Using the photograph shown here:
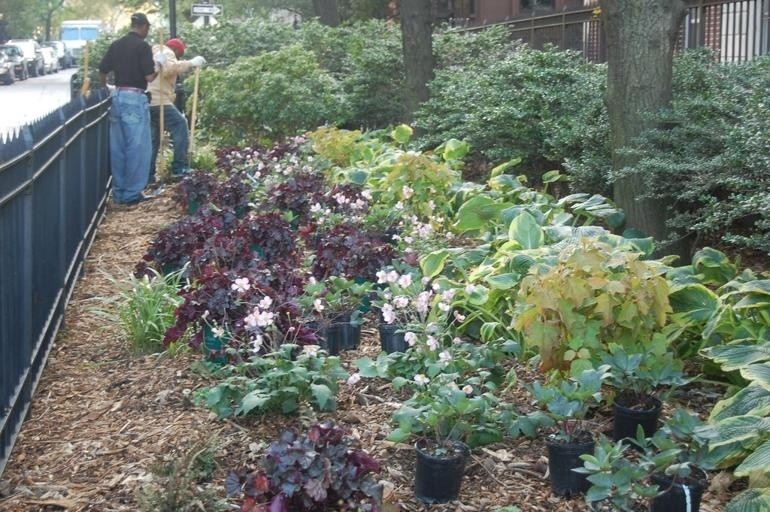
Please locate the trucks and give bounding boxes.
[57,19,104,69]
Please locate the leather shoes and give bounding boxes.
[128,191,154,205]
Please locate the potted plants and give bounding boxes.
[154,173,734,511]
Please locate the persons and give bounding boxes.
[145,38,207,184]
[99,13,167,206]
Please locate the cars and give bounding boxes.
[0,35,68,85]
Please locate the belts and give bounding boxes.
[115,87,146,94]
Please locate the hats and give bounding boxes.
[131,13,151,25]
[166,39,184,56]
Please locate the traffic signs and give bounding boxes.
[192,4,223,17]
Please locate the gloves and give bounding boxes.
[190,56,206,66]
[157,54,166,64]
